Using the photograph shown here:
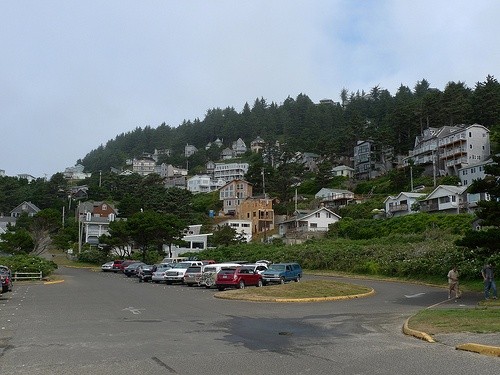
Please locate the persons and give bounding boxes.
[151,265,157,283]
[481,260,499,301]
[0,271,5,295]
[447,266,460,298]
[138,265,144,283]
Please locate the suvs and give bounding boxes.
[215,266,263,291]
[261,262,303,286]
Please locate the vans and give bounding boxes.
[202,263,240,289]
[163,260,203,285]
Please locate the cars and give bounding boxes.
[183,264,208,286]
[159,257,273,276]
[151,266,172,284]
[101,259,157,282]
